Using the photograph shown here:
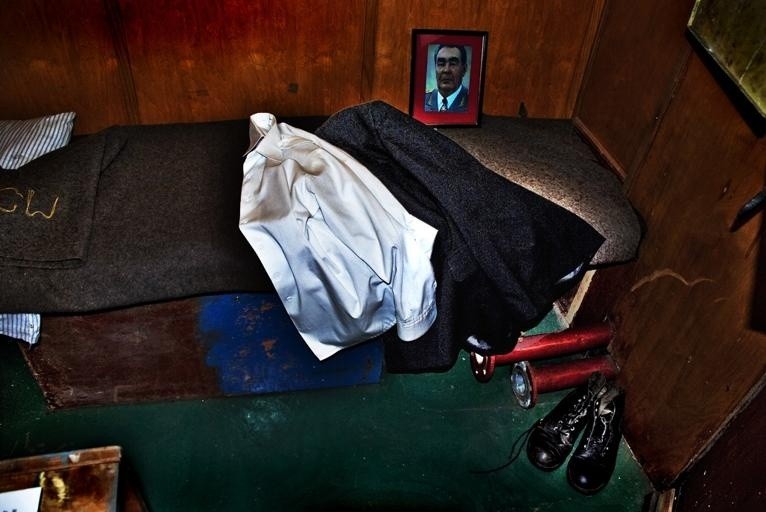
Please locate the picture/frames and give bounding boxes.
[409,26,487,128]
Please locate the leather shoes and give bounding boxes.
[567,383,626,494]
[527,370,606,471]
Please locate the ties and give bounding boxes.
[440,99,448,112]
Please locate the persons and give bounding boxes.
[423,43,470,114]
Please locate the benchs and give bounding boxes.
[1,116,644,328]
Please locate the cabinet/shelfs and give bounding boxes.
[0,446,150,512]
